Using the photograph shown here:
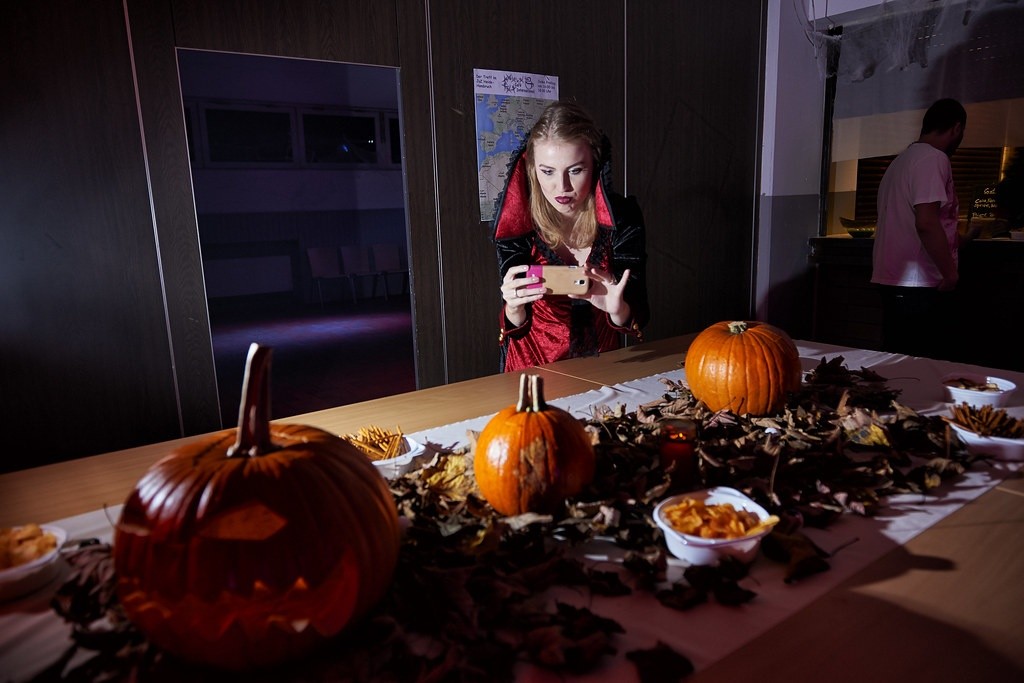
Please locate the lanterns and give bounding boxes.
[109,341,400,672]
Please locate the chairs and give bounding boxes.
[371,242,409,303]
[308,246,356,315]
[342,245,387,309]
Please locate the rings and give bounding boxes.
[514,288,519,298]
[609,273,617,285]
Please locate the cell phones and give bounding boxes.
[525,265,590,295]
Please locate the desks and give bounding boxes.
[0,332,1023,683]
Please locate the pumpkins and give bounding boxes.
[685,319,804,417]
[474,372,596,519]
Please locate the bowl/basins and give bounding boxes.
[0,526,68,593]
[371,436,427,480]
[655,485,771,568]
[938,372,1016,408]
[950,422,1024,462]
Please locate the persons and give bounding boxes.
[859,96,967,353]
[489,101,650,371]
